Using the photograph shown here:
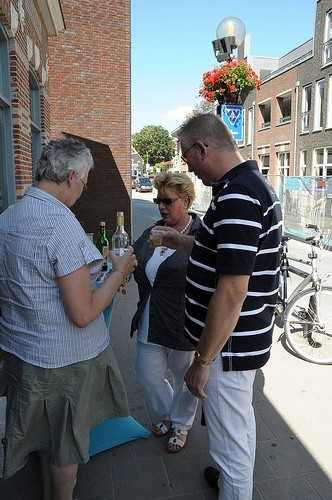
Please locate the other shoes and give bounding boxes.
[204,466,219,492]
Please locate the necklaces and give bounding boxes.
[160,215,192,256]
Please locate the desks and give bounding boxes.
[87,263,150,456]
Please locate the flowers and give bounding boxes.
[195,59,261,105]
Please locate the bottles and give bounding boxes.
[112,211,130,283]
[95,222,109,270]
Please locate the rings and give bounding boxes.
[134,261,137,266]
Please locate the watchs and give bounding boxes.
[194,350,214,365]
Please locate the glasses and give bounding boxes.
[153,197,178,205]
[180,141,208,163]
[80,179,88,193]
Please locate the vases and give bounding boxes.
[214,86,254,105]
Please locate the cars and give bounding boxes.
[131,175,138,188]
[136,177,154,192]
[148,176,155,182]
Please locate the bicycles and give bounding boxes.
[277,224,332,365]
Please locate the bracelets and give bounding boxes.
[112,269,126,282]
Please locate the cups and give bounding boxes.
[153,229,162,245]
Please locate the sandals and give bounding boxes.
[167,428,188,452]
[154,416,172,435]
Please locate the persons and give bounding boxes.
[146,115,283,500]
[131,173,202,451]
[0,138,137,500]
